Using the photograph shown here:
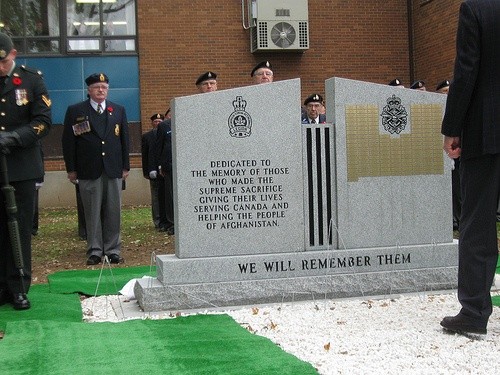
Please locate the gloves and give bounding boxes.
[35,182,43,190]
[150,170,157,178]
[0,131,21,147]
[72,180,78,184]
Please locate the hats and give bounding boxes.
[150,114,164,122]
[196,71,217,86]
[251,61,272,78]
[86,73,108,86]
[0,33,13,60]
[304,94,324,106]
[165,108,171,116]
[436,80,450,91]
[389,79,403,86]
[410,80,425,89]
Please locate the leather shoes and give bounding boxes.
[11,293,30,310]
[109,253,120,264]
[87,254,101,265]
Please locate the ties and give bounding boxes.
[0,75,9,89]
[97,104,103,114]
[311,120,316,124]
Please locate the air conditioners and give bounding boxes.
[247,0,309,52]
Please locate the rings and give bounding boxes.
[446,153,449,156]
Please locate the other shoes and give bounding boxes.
[168,229,174,235]
[159,225,167,231]
[80,232,87,240]
[32,228,38,236]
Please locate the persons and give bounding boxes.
[251,60,273,84]
[32,20,43,50]
[301,94,326,123]
[62,74,131,264]
[194,72,219,93]
[388,78,404,87]
[77,90,173,237]
[0,33,51,311]
[410,81,426,90]
[435,80,450,93]
[441,0,500,334]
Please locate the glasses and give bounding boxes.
[200,81,217,88]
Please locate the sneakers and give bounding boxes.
[441,312,488,331]
